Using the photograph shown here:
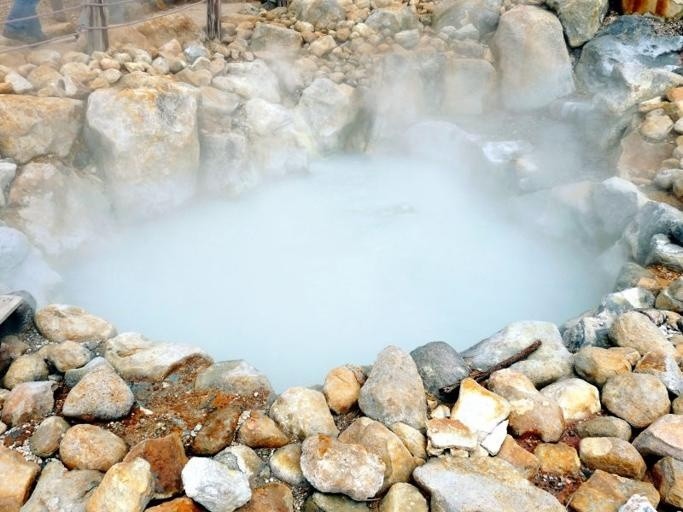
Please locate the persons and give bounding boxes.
[1,0,50,43]
